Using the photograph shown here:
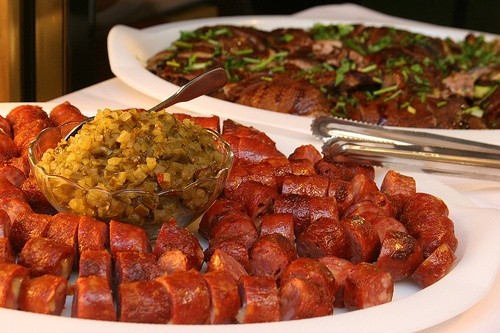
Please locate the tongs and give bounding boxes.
[310,114,500,170]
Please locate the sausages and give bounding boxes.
[0,100,458,325]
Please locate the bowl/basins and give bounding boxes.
[27,118,234,242]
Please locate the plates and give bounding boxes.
[0,109,499,332]
[107,2,500,149]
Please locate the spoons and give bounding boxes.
[62,68,228,144]
